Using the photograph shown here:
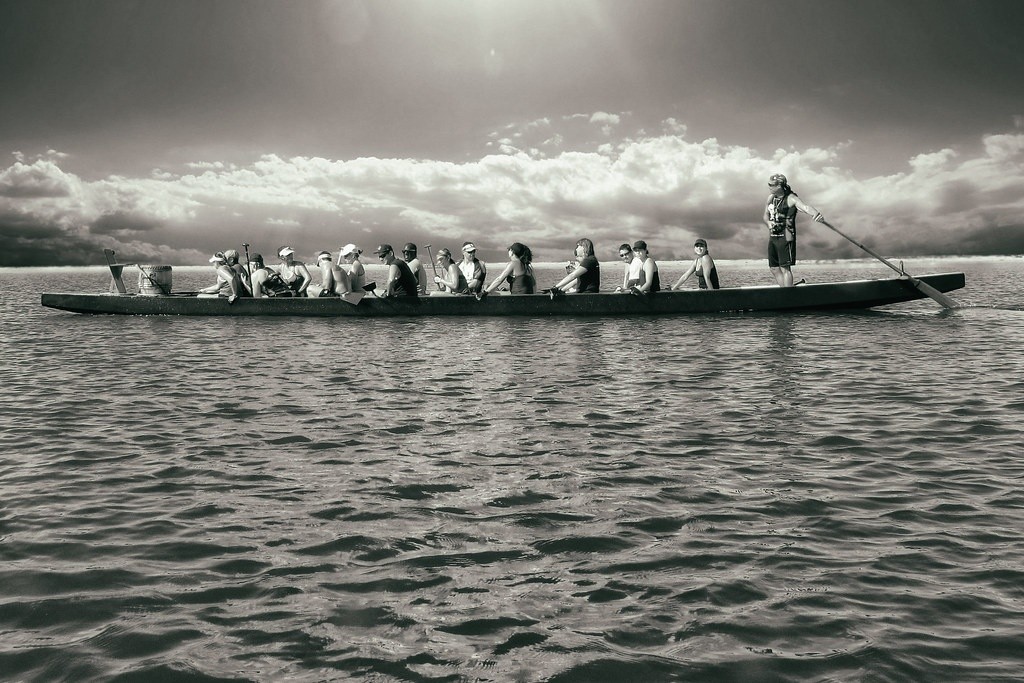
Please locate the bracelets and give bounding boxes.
[439,278,441,282]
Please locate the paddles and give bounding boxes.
[423,244,441,291]
[821,219,959,309]
[242,242,253,296]
[326,289,365,306]
[615,286,646,297]
[361,282,377,297]
[793,279,805,285]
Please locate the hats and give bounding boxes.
[245,253,263,263]
[340,244,360,257]
[209,254,224,263]
[373,244,393,253]
[316,254,332,267]
[462,244,477,253]
[693,238,707,245]
[225,250,239,259]
[632,241,646,250]
[279,247,297,258]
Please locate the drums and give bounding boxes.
[138,263,172,294]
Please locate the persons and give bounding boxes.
[541,238,660,296]
[433,241,537,301]
[671,239,719,290]
[763,173,824,288]
[196,243,427,304]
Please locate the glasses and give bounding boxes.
[379,252,389,259]
[768,183,776,187]
[401,249,415,255]
[620,252,630,257]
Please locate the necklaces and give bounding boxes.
[773,196,783,212]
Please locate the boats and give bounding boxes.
[40,248,967,317]
